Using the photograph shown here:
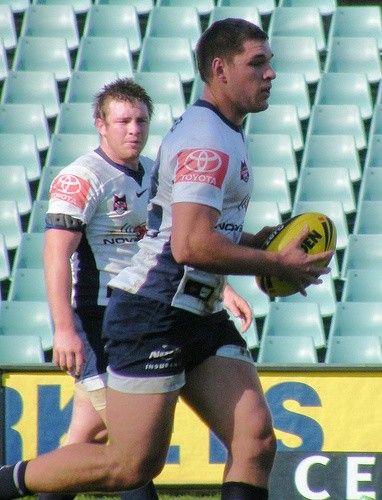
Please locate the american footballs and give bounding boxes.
[255,212,337,297]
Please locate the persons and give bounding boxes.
[0,18,335,500]
[38,78,253,500]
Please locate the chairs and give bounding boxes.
[0,0,382,367]
